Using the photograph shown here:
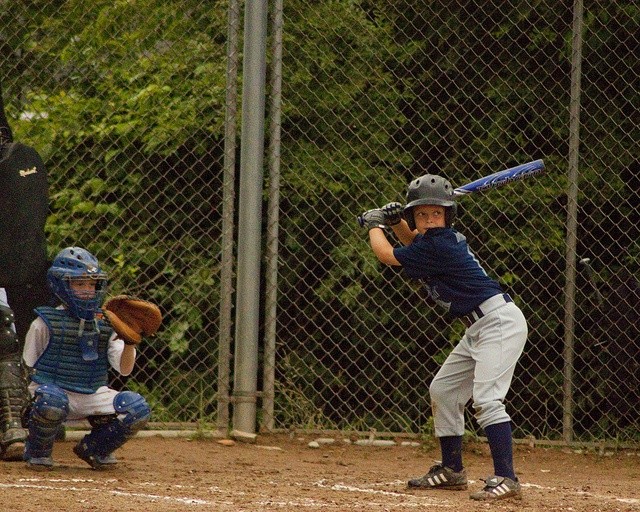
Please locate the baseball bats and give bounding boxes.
[357,158,546,228]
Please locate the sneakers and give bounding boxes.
[0,441,26,459]
[26,455,53,472]
[468,474,521,501]
[73,440,120,471]
[408,464,468,491]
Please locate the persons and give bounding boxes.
[0,137,66,441]
[22,246,148,470]
[360,176,529,500]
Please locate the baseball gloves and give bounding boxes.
[101,294,163,345]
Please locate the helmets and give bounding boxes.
[403,173,458,232]
[46,246,107,321]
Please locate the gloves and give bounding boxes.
[361,207,386,233]
[381,201,404,226]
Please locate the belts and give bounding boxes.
[459,292,513,328]
